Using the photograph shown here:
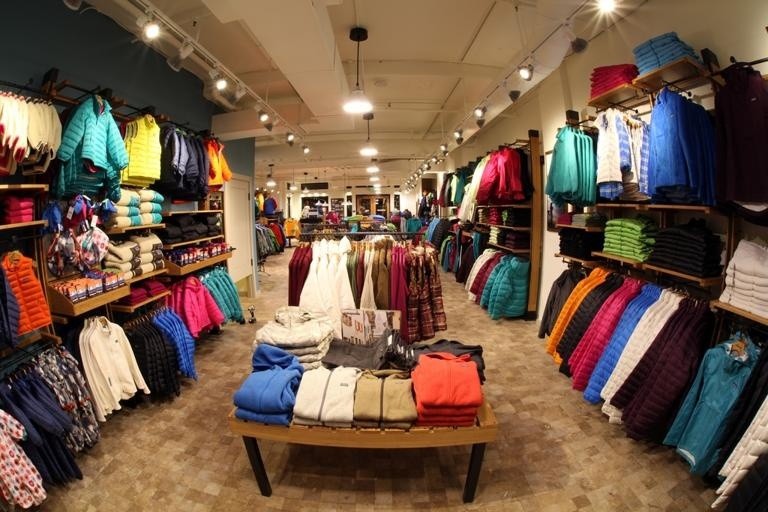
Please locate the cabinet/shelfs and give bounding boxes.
[633,56,712,94]
[94,209,233,314]
[474,204,530,255]
[587,81,647,109]
[548,204,730,287]
[0,178,52,234]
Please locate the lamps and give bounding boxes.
[342,25,372,116]
[358,114,377,157]
[135,8,160,39]
[454,126,464,145]
[440,144,449,157]
[178,38,195,60]
[475,106,487,129]
[507,63,536,105]
[253,105,280,133]
[563,25,588,53]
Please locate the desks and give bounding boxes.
[226,389,499,503]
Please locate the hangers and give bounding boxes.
[654,81,695,106]
[598,103,641,125]
[555,123,597,139]
